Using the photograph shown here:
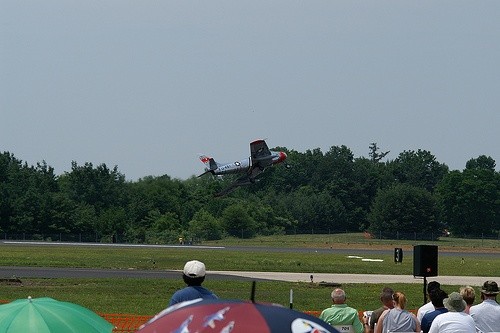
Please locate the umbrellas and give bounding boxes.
[0,294,114,333]
[136,299,340,333]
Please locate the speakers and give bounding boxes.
[413,245,438,276]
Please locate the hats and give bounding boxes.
[443,291,467,311]
[481,281,500,295]
[182,260,206,278]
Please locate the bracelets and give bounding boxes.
[363,323,368,328]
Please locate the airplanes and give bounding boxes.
[195,138,288,199]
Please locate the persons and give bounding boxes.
[319,288,370,333]
[370,288,394,333]
[470,280,500,333]
[169,259,218,307]
[421,290,449,333]
[377,292,421,333]
[459,286,476,313]
[428,292,478,333]
[416,281,440,333]
